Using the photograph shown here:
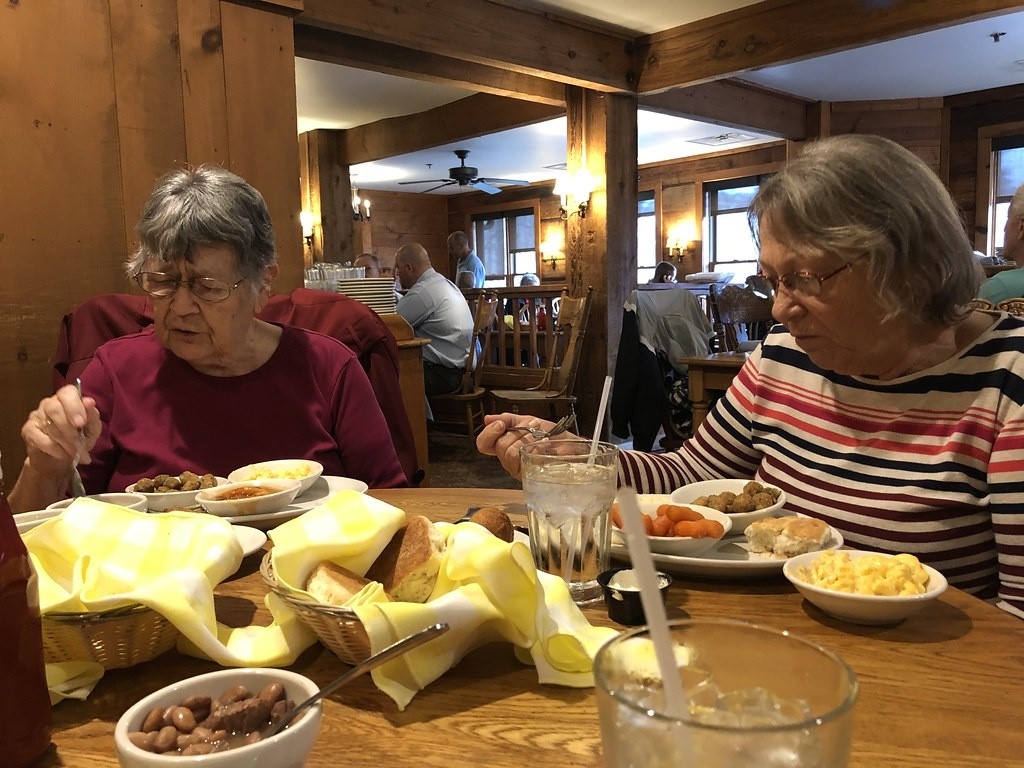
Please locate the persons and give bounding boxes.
[504,272,543,320]
[477,133,1024,619]
[392,243,484,392]
[382,267,402,290]
[0,163,407,516]
[456,270,478,309]
[975,184,1024,304]
[646,260,678,285]
[354,253,404,304]
[447,230,485,289]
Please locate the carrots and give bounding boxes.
[611,502,724,539]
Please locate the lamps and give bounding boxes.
[543,250,565,269]
[300,212,316,248]
[667,232,688,261]
[553,171,597,219]
[351,188,371,221]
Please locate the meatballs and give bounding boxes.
[692,481,781,511]
[133,471,218,493]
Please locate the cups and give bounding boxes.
[597,565,672,624]
[519,439,620,607]
[592,616,858,768]
[304,262,366,291]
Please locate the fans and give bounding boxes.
[398,150,534,195]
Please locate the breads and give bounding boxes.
[742,515,832,557]
[304,513,448,608]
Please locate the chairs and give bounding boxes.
[50,286,413,489]
[490,283,596,438]
[709,280,773,351]
[431,291,502,442]
[611,288,716,455]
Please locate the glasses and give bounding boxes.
[132,258,252,301]
[745,250,873,300]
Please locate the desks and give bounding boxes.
[12,486,1024,768]
[395,338,435,474]
[675,350,752,440]
[478,328,569,368]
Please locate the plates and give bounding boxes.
[217,475,368,530]
[595,494,845,581]
[512,530,530,548]
[232,525,267,557]
[335,277,397,315]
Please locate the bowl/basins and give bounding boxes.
[46,492,148,513]
[113,667,322,768]
[194,477,302,516]
[671,478,788,534]
[229,459,324,497]
[612,502,733,558]
[782,549,949,625]
[13,508,66,533]
[125,475,229,511]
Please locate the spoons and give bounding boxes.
[71,377,85,498]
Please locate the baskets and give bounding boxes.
[261,548,371,667]
[40,602,174,671]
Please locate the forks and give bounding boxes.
[505,414,577,439]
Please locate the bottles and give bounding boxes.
[536,307,547,330]
[0,461,61,768]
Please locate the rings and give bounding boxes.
[40,420,54,430]
